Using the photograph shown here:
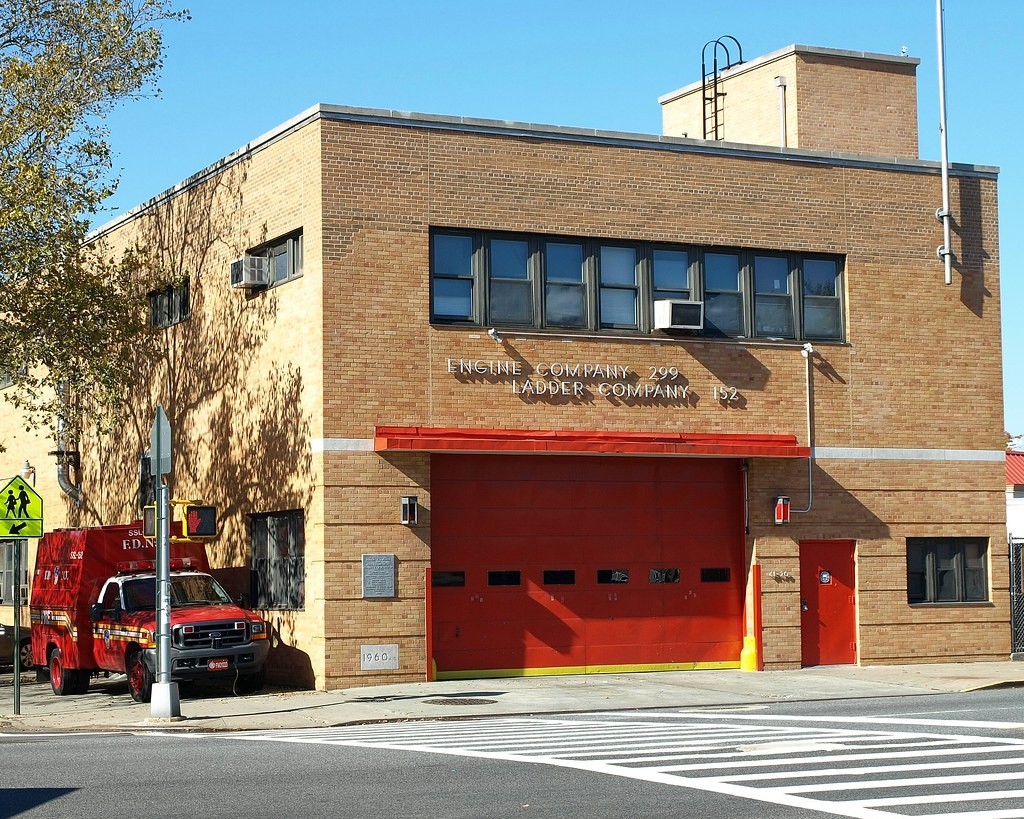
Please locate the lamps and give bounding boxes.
[22,460,35,479]
[401,493,418,524]
[774,495,791,525]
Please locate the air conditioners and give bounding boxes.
[231,256,268,287]
[654,298,704,329]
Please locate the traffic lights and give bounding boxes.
[181,505,218,540]
[142,501,162,540]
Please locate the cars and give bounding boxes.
[0,623,37,671]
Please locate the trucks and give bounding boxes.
[28,520,272,704]
